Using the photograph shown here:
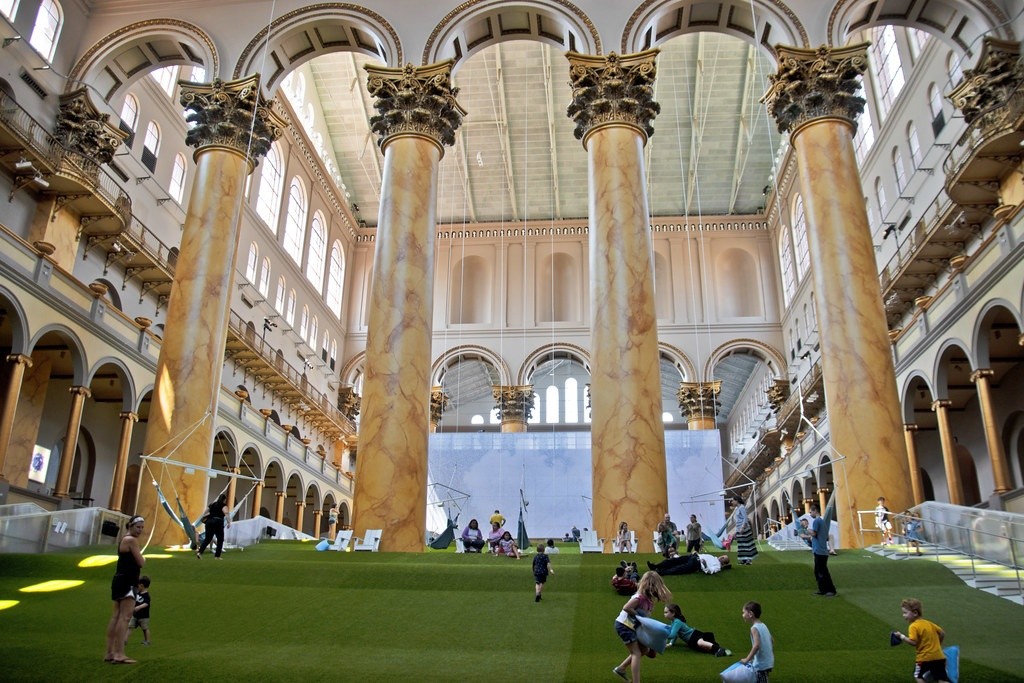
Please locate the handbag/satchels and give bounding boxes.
[201,512,210,524]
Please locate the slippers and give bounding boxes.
[104,657,136,665]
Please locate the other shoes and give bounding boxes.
[535,592,541,602]
[140,640,151,646]
[829,551,837,556]
[814,592,837,597]
[197,553,202,559]
[620,560,637,572]
[214,557,224,560]
[646,560,657,571]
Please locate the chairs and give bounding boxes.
[453,528,477,554]
[320,530,353,552]
[650,531,663,553]
[351,529,382,552]
[612,531,639,554]
[578,531,606,554]
[487,541,492,552]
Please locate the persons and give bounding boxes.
[733,495,759,565]
[657,514,680,558]
[124,575,151,646]
[739,601,774,683]
[647,551,729,575]
[806,504,837,597]
[104,516,145,664]
[663,604,732,658]
[875,497,895,546]
[571,526,580,542]
[544,539,559,554]
[532,544,555,602]
[490,510,506,528]
[612,561,640,596]
[195,494,230,560]
[798,518,838,557]
[498,531,524,560]
[488,522,505,554]
[901,509,926,558]
[686,514,702,553]
[894,598,951,683]
[462,518,485,553]
[612,571,671,683]
[617,522,632,553]
[327,503,340,541]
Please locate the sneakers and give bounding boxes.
[613,667,629,682]
[715,647,732,658]
[738,562,752,566]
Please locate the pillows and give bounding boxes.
[315,539,329,551]
[922,644,961,683]
[635,615,672,655]
[720,660,759,683]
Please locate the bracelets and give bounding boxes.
[899,634,906,641]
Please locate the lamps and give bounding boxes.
[331,165,359,214]
[882,225,896,239]
[763,140,787,196]
[801,351,810,360]
